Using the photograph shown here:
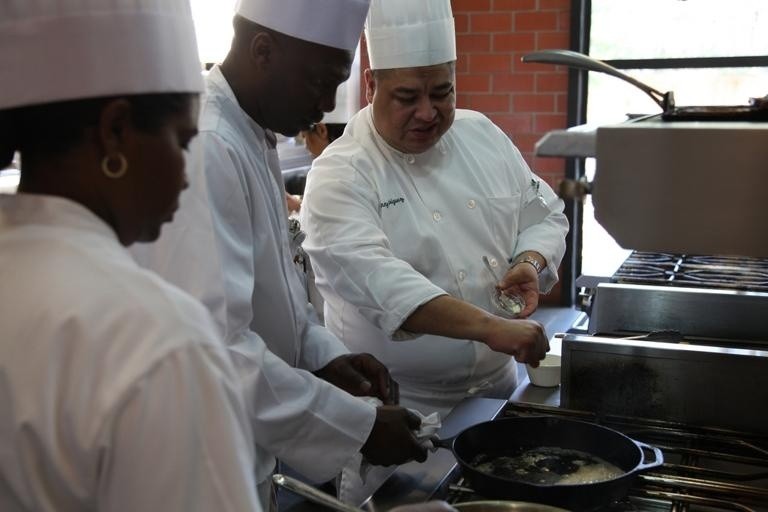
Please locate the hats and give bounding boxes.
[235,0,368,53]
[0,0,208,114]
[362,0,457,72]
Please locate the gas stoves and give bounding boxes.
[432,395,768,510]
[609,249,766,294]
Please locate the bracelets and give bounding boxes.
[507,256,543,274]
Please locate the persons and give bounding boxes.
[119,10,429,512]
[295,61,574,507]
[285,122,347,218]
[1,91,264,512]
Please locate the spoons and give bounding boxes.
[481,250,527,320]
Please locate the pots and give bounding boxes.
[384,411,664,502]
[520,41,764,118]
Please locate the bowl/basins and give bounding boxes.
[524,349,565,386]
